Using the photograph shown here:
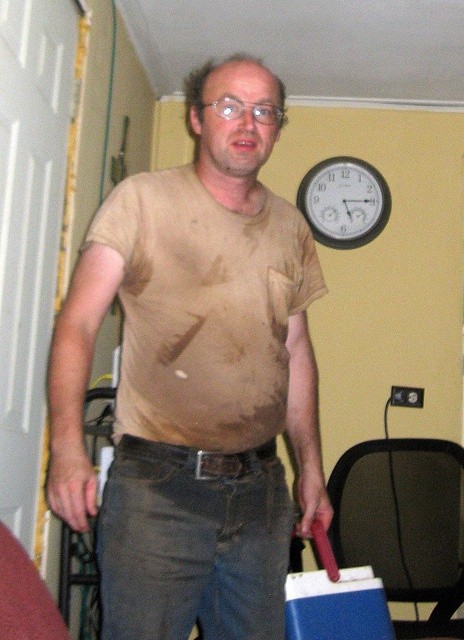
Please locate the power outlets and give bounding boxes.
[390,386,425,408]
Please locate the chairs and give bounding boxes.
[324,439,463,639]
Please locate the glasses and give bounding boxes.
[202,94,287,125]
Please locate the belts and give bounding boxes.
[116,433,276,483]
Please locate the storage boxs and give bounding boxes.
[279,514,398,638]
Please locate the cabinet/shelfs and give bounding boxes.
[57,386,119,640]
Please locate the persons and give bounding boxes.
[44,54,333,639]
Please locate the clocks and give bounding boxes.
[298,156,392,249]
